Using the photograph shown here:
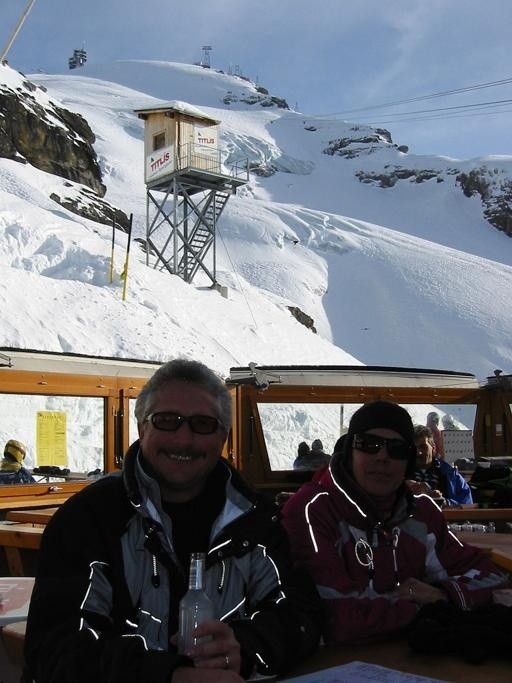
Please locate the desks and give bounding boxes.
[275,634,512,683]
[0,506,60,673]
[440,506,512,572]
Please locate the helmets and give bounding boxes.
[4,439,25,463]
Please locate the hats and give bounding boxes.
[349,401,415,439]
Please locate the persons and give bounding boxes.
[425,411,443,460]
[24,358,322,683]
[440,414,460,430]
[277,399,512,646]
[401,425,473,508]
[0,439,37,486]
[299,439,332,471]
[293,441,310,469]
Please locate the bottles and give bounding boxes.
[176,552,217,664]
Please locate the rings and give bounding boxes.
[224,654,230,667]
[409,587,415,596]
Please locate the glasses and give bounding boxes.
[142,411,226,435]
[352,432,411,460]
